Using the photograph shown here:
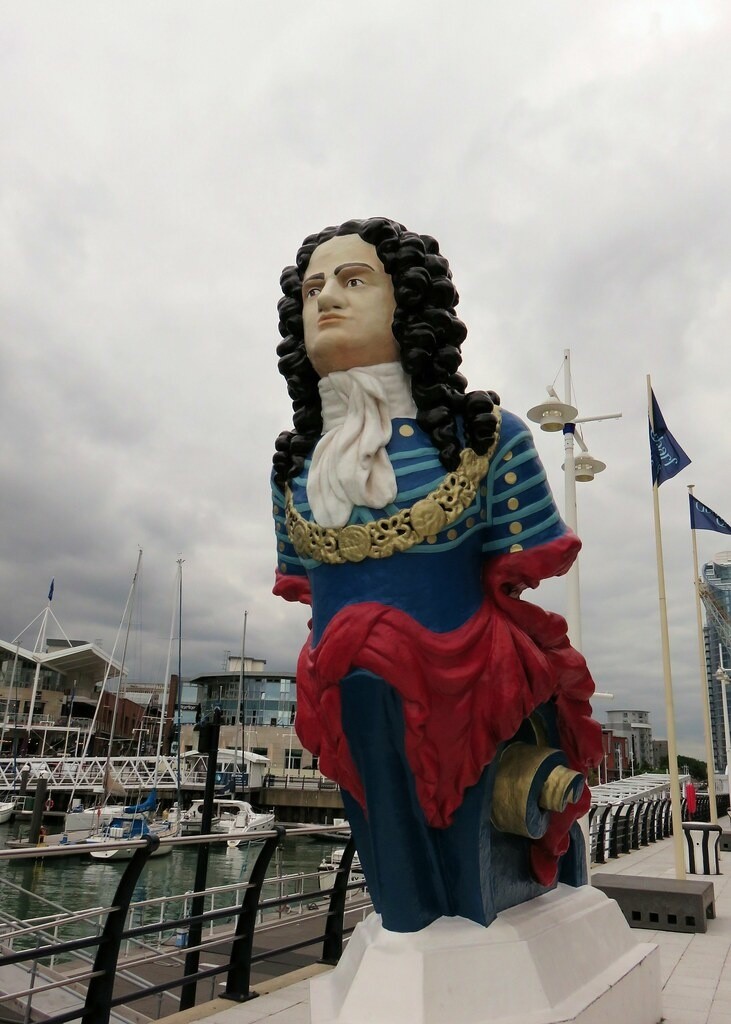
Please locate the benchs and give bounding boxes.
[591,872,716,934]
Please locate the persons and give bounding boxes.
[267,216,605,936]
[162,807,169,821]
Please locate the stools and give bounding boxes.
[682,821,722,874]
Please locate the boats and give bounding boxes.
[0,542,367,890]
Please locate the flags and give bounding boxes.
[647,383,692,491]
[689,493,731,535]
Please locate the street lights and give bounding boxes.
[527,348,625,680]
